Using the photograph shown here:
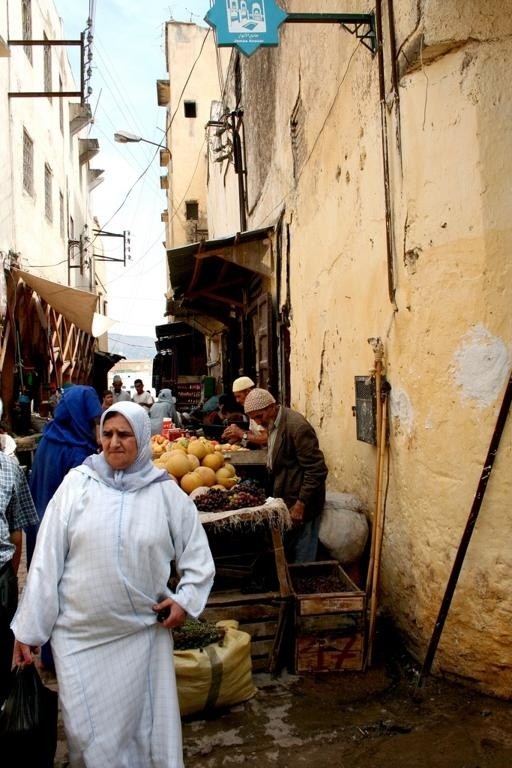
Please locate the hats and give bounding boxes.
[232,377,255,393]
[244,387,276,412]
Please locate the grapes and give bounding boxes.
[194,484,266,513]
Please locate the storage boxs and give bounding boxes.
[199,561,368,674]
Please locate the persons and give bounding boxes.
[1,450,39,672]
[184,382,249,440]
[11,400,217,768]
[23,383,104,675]
[12,386,64,471]
[99,375,184,438]
[232,377,329,562]
